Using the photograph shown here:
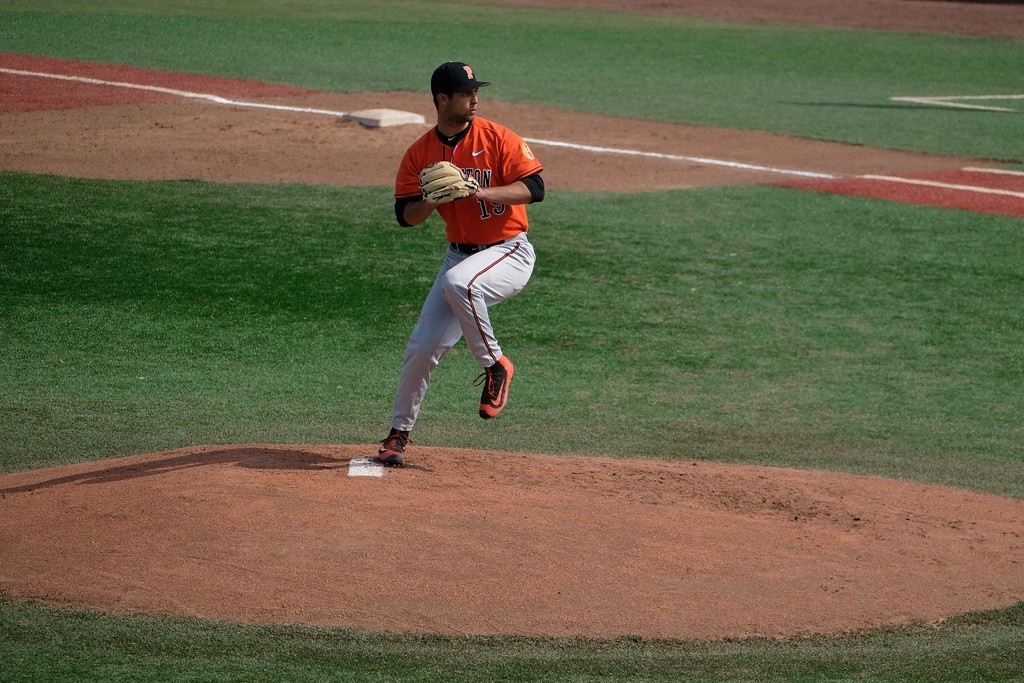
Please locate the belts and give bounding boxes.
[450,239,505,254]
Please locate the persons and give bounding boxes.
[377,60,545,465]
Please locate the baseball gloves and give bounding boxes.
[417,160,480,204]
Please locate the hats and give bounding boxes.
[431,61,492,97]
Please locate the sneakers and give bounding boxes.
[378,430,412,468]
[479,354,514,419]
